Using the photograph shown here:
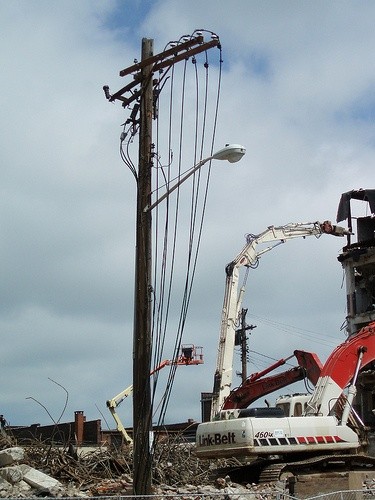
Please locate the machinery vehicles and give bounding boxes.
[195,221,373,487]
[106,344,203,455]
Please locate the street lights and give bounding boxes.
[101,37,245,500]
